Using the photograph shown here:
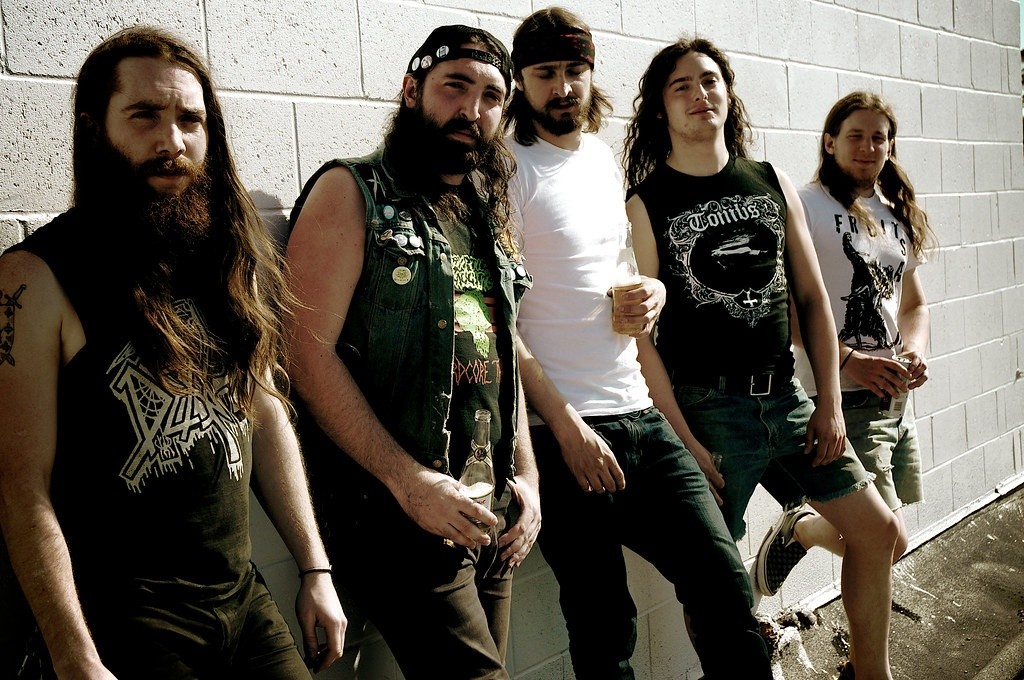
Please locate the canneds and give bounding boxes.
[879,355,913,418]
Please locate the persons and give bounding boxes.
[282,25,542,680]
[621,38,900,680]
[500,7,779,680]
[1,29,347,680]
[757,91,932,599]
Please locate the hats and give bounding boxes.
[406,23,514,101]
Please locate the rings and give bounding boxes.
[443,539,456,549]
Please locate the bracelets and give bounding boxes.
[299,565,333,579]
[840,349,855,371]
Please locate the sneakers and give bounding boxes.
[756,507,816,597]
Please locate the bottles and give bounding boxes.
[458,410,496,535]
[612,222,645,333]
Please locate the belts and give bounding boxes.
[580,407,648,425]
[736,371,789,398]
